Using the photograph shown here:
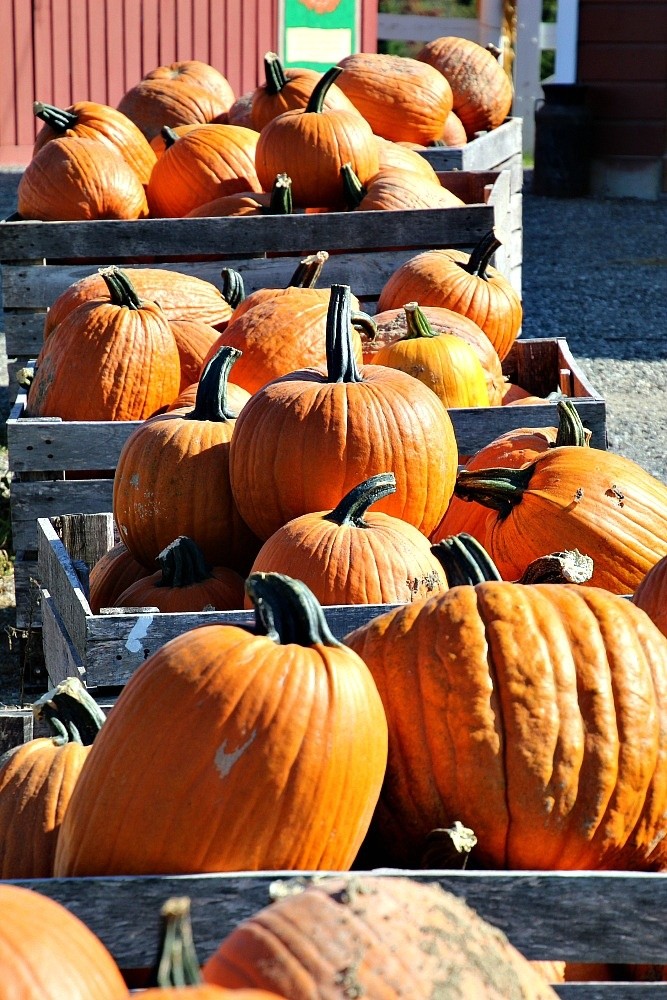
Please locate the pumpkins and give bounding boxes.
[1,228,667,1000]
[16,36,513,265]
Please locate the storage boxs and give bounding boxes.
[1,118,667,1000]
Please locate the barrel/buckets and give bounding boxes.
[532,84,596,198]
